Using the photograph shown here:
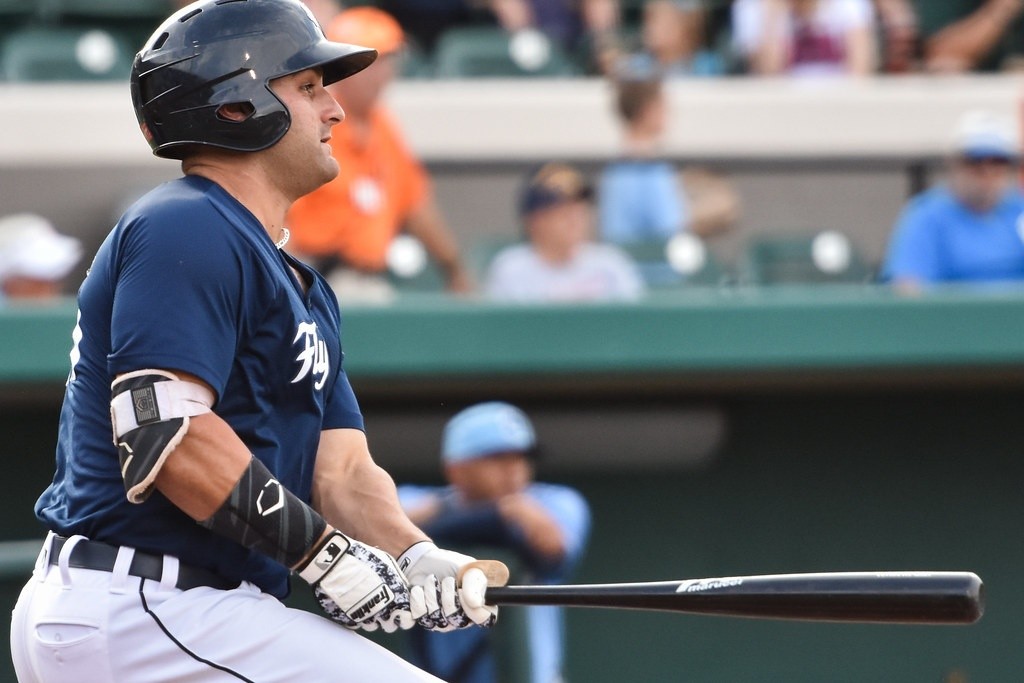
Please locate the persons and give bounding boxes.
[396,402,588,683]
[280,0,1024,306]
[0,212,83,299]
[9,0,508,683]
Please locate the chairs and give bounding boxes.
[0,1,1024,287]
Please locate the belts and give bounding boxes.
[49,536,239,592]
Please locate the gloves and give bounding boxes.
[293,528,416,635]
[396,540,510,632]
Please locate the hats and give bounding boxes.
[516,161,595,217]
[321,6,405,58]
[958,136,1015,163]
[441,400,537,465]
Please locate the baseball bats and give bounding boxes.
[412,570,986,625]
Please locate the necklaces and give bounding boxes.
[275,228,290,250]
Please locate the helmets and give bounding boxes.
[130,0,379,160]
